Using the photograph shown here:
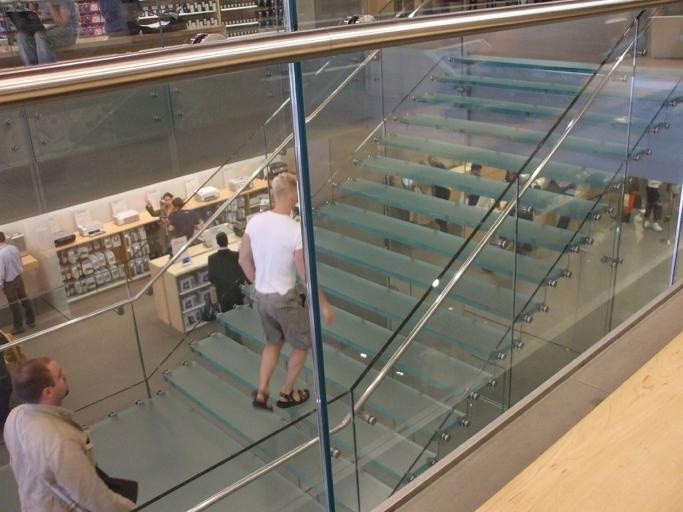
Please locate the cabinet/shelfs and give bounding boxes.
[55,176,269,333]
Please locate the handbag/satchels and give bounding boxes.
[210,286,217,304]
[96,467,137,503]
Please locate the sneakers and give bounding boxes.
[644,221,662,232]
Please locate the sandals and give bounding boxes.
[253,389,309,411]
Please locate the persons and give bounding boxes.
[206,232,253,346]
[14,0,77,67]
[166,197,201,247]
[423,157,681,274]
[0,233,36,334]
[96,0,143,38]
[235,173,337,410]
[142,192,175,254]
[3,356,139,512]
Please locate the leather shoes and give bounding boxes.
[11,323,35,335]
